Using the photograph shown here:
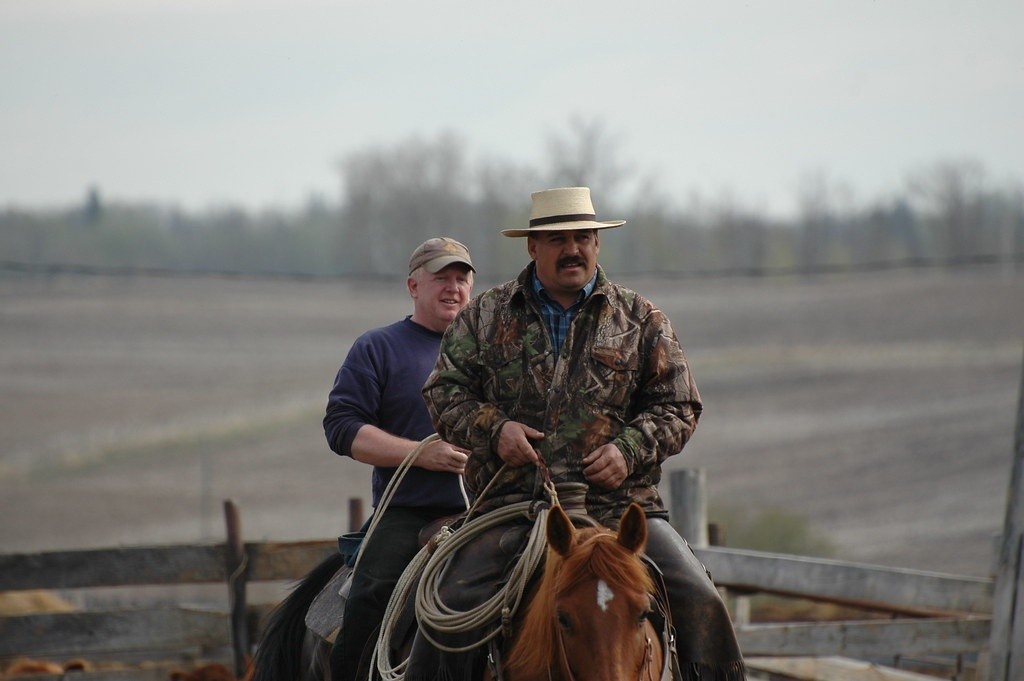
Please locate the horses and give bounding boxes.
[252,503,678,680]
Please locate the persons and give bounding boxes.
[404,187,746,681]
[323,236,478,681]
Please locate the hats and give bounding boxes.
[500,187,626,237]
[408,238,477,274]
